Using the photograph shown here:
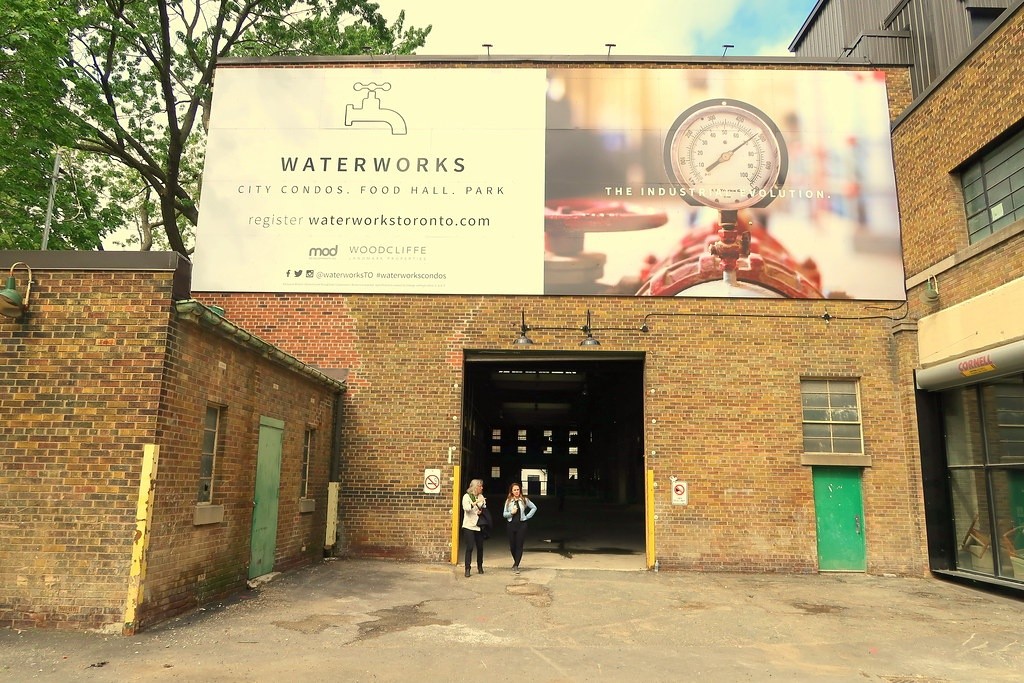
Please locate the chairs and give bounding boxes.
[960,515,1024,580]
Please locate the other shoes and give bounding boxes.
[512,566,520,573]
[479,567,484,574]
[465,568,470,578]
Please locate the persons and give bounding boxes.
[462,479,486,577]
[503,483,537,574]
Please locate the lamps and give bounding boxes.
[0,262,33,319]
[919,272,940,305]
[580,309,601,346]
[512,311,534,346]
